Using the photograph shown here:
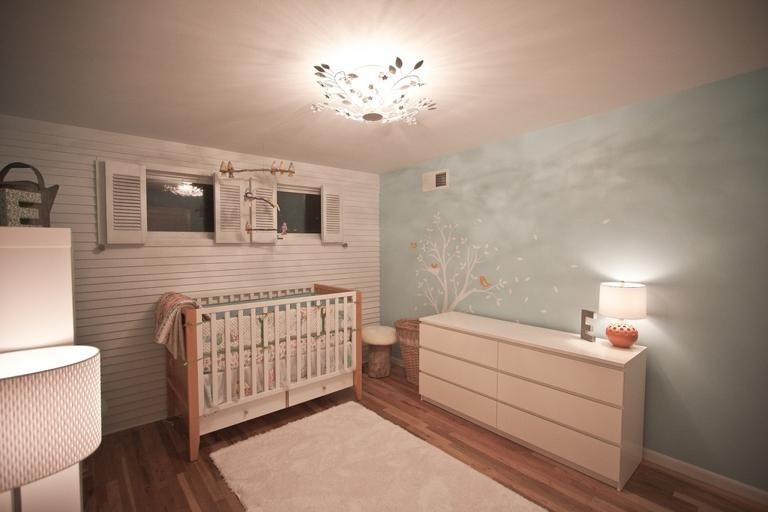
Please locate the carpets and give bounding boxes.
[210,399,551,512]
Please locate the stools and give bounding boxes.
[363,325,398,377]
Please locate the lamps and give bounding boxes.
[310,56,438,126]
[0,345,103,511]
[597,279,649,348]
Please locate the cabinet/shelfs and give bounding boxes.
[418,310,649,490]
[0,226,85,512]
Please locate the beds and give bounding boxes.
[151,282,364,462]
